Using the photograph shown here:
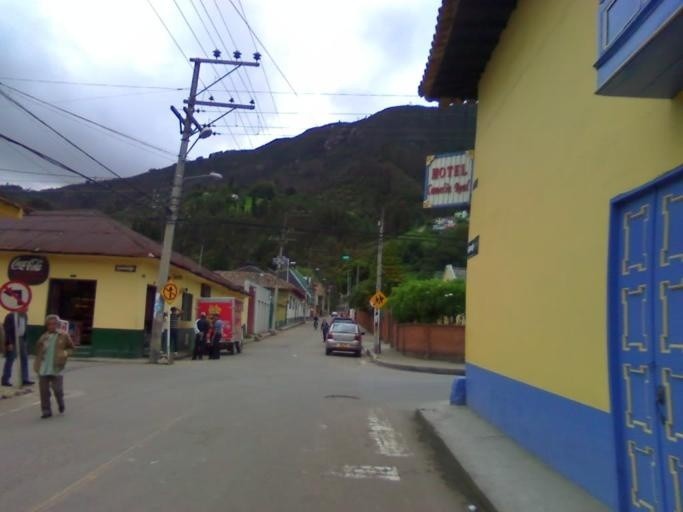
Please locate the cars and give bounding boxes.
[329,316,352,328]
[331,311,338,316]
[326,323,366,355]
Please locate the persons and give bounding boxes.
[321,318,329,342]
[33,314,74,418]
[1,304,36,386]
[161,307,224,360]
[313,316,319,327]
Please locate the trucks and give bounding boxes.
[196,297,246,355]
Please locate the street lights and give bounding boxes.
[146,172,222,362]
[301,267,326,325]
[269,261,297,332]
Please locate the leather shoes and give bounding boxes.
[42,413,51,418]
[1,381,12,386]
[21,380,35,385]
